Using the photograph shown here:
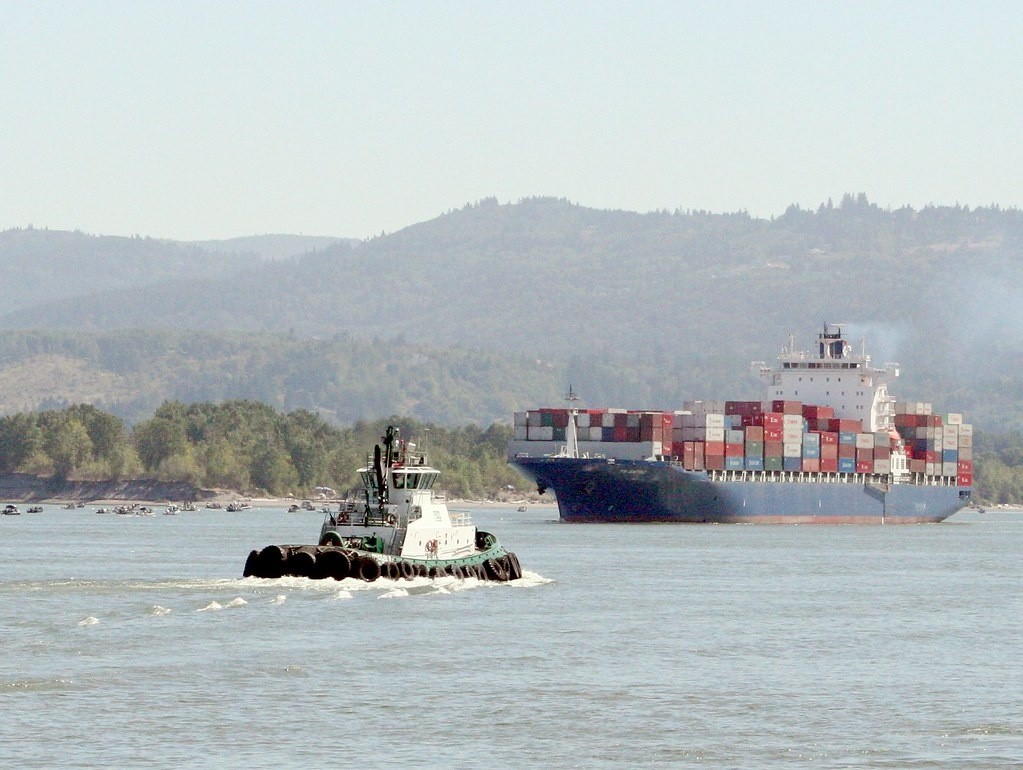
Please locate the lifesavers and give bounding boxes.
[318,531,343,546]
[242,546,521,582]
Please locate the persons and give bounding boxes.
[363,504,375,523]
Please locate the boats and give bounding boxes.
[2,505,20,516]
[978,508,986,513]
[507,320,976,526]
[77,501,84,507]
[164,507,181,515]
[114,504,156,516]
[288,504,301,512]
[243,424,521,583]
[205,501,251,511]
[62,503,74,509]
[28,505,44,513]
[181,503,200,511]
[301,501,332,513]
[96,509,111,514]
